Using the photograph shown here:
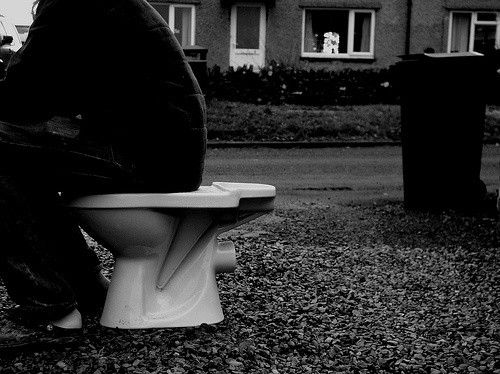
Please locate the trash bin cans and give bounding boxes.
[395,51,487,215]
[181,46,209,105]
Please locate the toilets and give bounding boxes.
[67,180,276,330]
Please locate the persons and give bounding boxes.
[1,0,208,339]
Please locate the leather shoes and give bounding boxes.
[0,274,110,359]
